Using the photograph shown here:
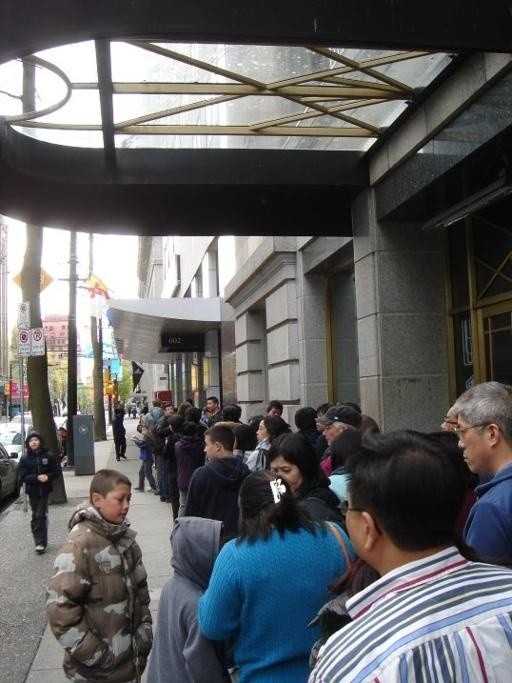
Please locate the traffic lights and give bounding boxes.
[105,386,112,395]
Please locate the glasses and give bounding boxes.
[337,499,383,536]
[455,424,487,435]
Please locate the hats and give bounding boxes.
[315,406,361,428]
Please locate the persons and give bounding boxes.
[127,380,512,682]
[15,429,62,553]
[46,469,153,683]
[112,406,129,463]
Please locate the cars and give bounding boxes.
[62,407,81,417]
[0,441,24,505]
[0,412,69,462]
